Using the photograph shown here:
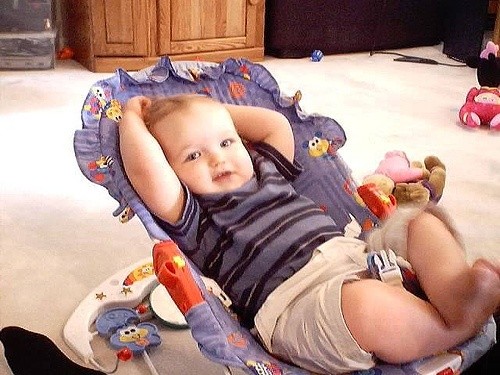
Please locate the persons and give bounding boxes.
[119,93,500,375]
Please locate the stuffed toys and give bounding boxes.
[362,150,446,208]
[459,87,500,127]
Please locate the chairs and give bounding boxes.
[62,57,496,375]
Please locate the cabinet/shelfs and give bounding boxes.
[60,0,265,72]
